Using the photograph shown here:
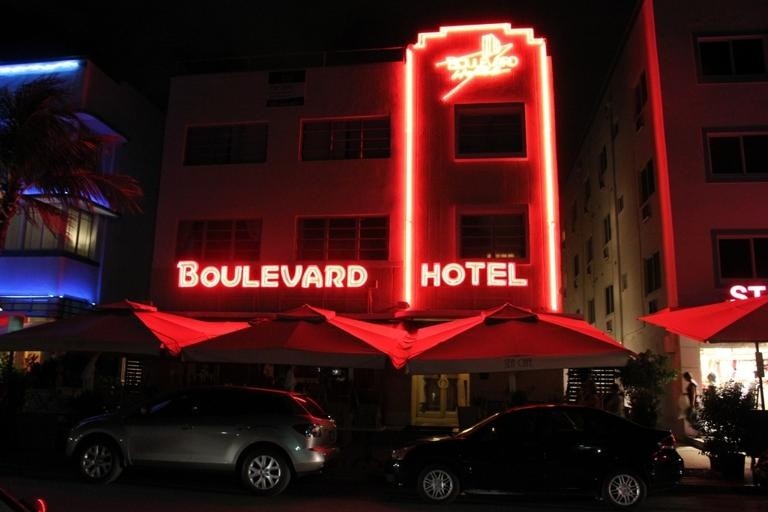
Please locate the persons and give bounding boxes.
[579,372,716,434]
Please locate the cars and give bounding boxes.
[382,402,684,507]
[755,449,768,489]
[0,485,47,512]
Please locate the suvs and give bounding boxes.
[64,385,339,497]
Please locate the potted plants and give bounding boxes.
[617,346,684,435]
[695,375,758,488]
